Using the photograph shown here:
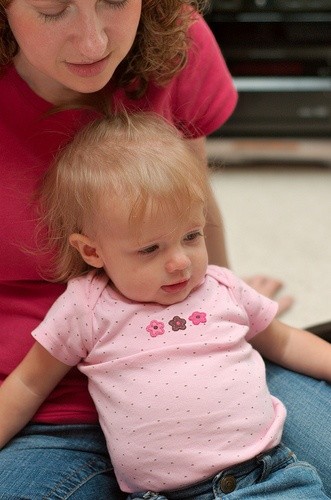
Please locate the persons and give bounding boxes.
[0,110,331,500]
[1,0,331,500]
